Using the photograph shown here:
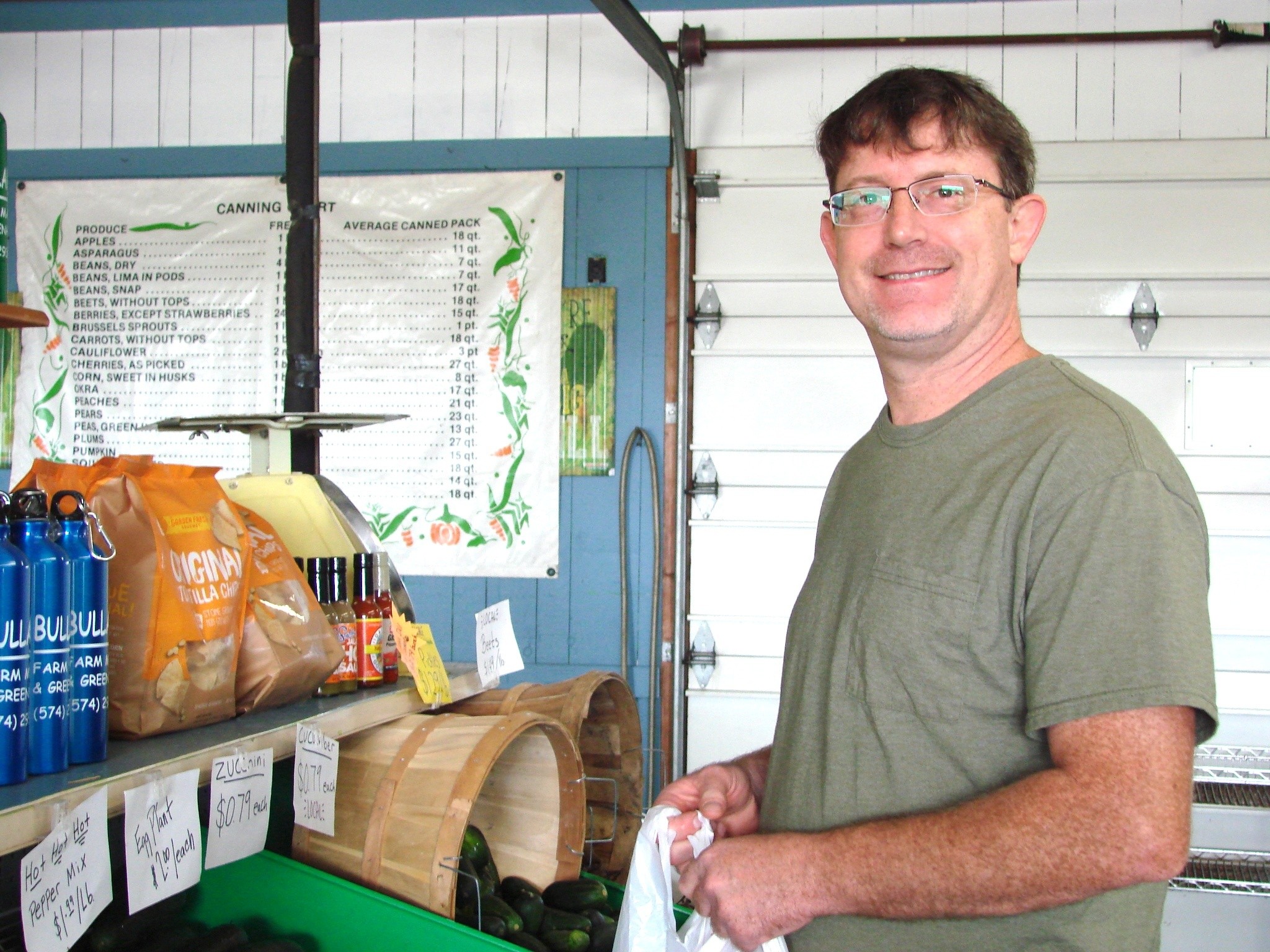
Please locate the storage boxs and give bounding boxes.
[194,774,692,952]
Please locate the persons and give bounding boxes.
[646,66,1216,952]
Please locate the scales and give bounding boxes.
[138,411,415,678]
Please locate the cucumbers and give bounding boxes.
[452,824,621,952]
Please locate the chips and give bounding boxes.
[155,660,189,714]
[212,498,244,550]
[186,638,233,691]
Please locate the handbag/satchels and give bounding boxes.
[612,803,789,952]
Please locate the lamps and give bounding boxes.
[690,622,716,690]
[695,278,724,350]
[691,451,717,519]
[1129,282,1158,354]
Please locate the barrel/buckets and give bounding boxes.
[444,669,642,889]
[293,710,587,922]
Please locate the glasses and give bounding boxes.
[821,174,1016,227]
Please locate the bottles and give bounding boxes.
[10,490,73,781]
[0,489,32,788]
[307,557,341,700]
[352,553,384,690]
[373,551,398,684]
[329,556,357,693]
[49,491,116,767]
